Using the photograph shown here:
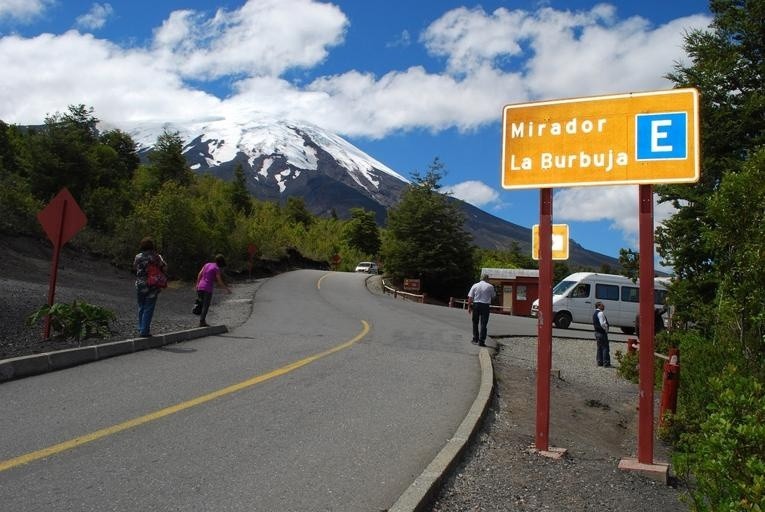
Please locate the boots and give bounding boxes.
[198,320,210,327]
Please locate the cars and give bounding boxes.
[355,262,378,273]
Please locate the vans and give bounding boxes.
[531,271,673,334]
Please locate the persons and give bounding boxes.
[467,273,496,347]
[197,254,231,327]
[636,314,639,339]
[593,302,615,368]
[133,237,168,338]
[654,304,668,334]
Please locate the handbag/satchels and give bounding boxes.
[190,293,205,316]
[146,262,169,290]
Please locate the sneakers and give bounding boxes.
[139,334,153,338]
[470,338,488,348]
